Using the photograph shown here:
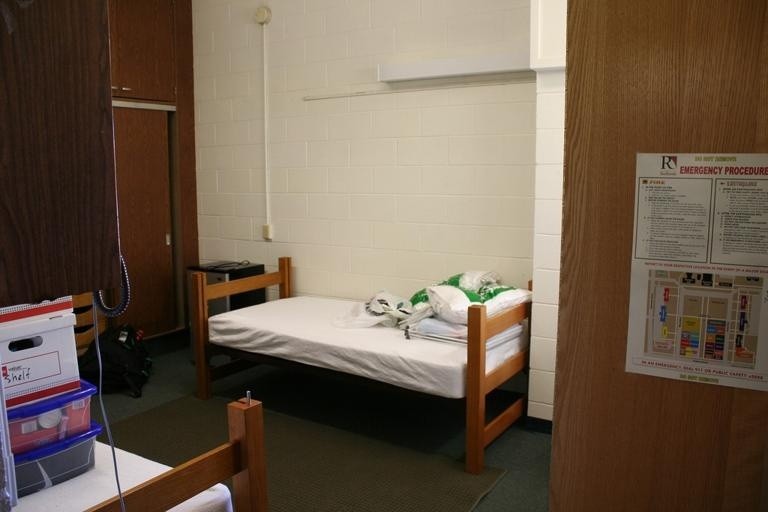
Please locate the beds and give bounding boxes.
[186,256,533,474]
[0,396,267,512]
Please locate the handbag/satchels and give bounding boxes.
[79,324,147,400]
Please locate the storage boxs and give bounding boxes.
[0,313,104,500]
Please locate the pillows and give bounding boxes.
[361,270,532,327]
